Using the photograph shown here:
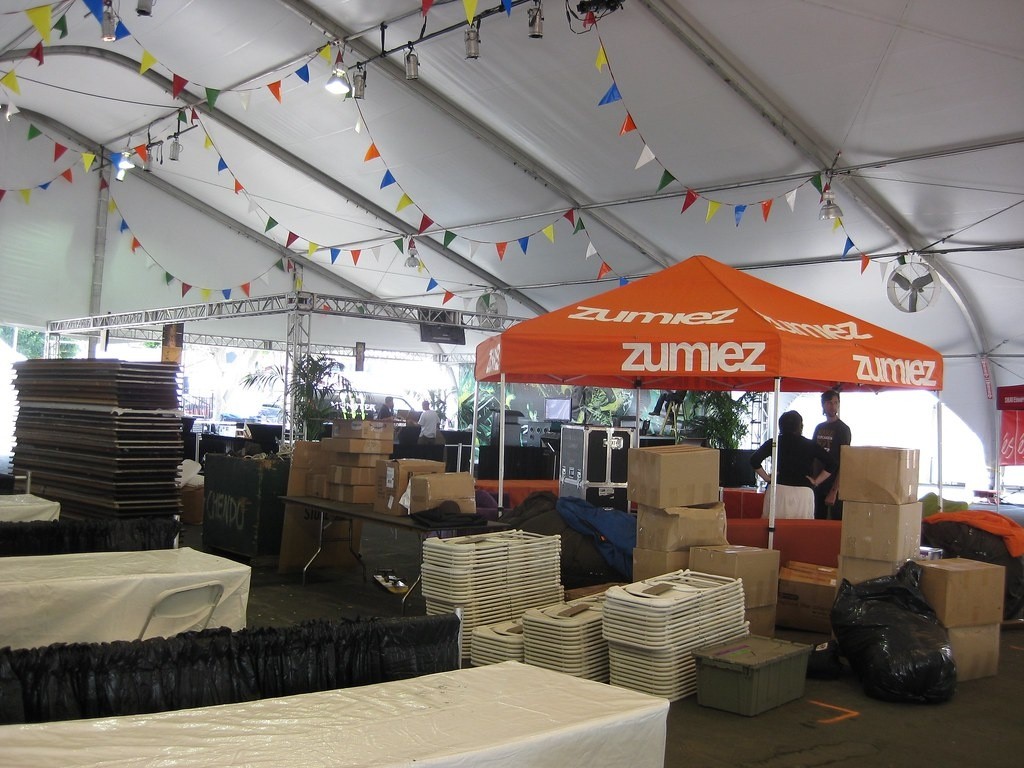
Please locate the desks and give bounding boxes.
[0,439,842,768]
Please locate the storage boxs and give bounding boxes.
[837,555,916,590]
[632,547,689,583]
[638,501,730,552]
[776,560,838,634]
[306,473,331,500]
[327,465,343,484]
[563,581,629,600]
[336,453,390,468]
[374,458,446,517]
[627,443,720,509]
[342,466,375,485]
[840,501,922,563]
[838,445,920,505]
[916,558,1006,629]
[330,483,345,501]
[321,438,393,454]
[689,545,780,610]
[332,418,394,441]
[410,472,478,515]
[946,623,1002,681]
[344,485,375,503]
[745,605,775,637]
[693,634,814,718]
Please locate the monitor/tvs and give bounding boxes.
[418,308,466,345]
[543,397,572,423]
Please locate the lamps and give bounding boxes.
[404,41,420,80]
[143,147,153,172]
[136,0,157,17]
[526,0,544,39]
[353,61,367,99]
[463,22,481,61]
[325,62,350,96]
[818,175,844,220]
[404,238,424,268]
[101,5,116,42]
[169,136,185,161]
[120,152,136,169]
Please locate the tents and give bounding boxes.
[469,256,944,550]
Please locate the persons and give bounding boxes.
[377,397,394,420]
[408,401,441,444]
[750,410,836,519]
[812,390,851,519]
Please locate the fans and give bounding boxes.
[475,289,508,326]
[887,254,941,314]
